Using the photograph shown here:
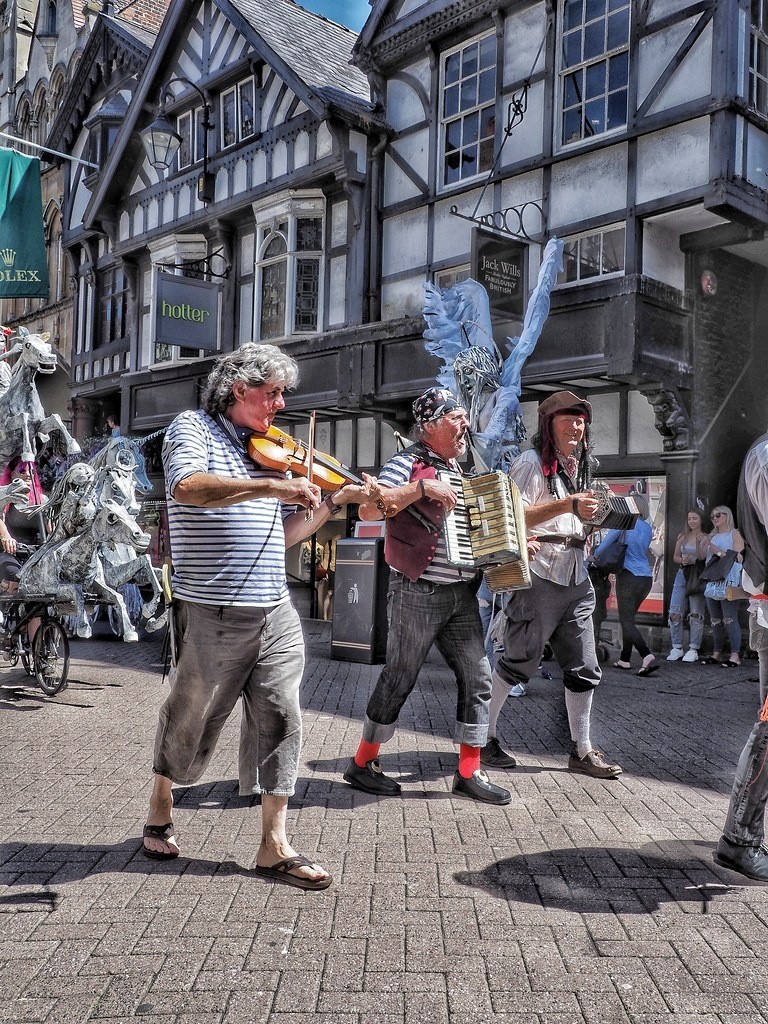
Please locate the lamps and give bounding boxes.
[139,76,214,203]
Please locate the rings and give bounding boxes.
[370,488,374,492]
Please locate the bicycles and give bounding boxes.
[0,542,115,695]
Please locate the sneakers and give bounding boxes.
[342,756,404,798]
[479,736,517,768]
[449,769,513,805]
[681,649,699,663]
[567,751,624,779]
[666,647,685,664]
[712,834,768,881]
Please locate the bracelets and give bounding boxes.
[593,556,596,560]
[420,479,425,498]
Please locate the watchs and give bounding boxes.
[716,551,722,556]
[324,494,342,517]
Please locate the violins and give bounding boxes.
[247,423,399,524]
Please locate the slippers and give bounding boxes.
[255,852,334,890]
[635,665,666,676]
[721,659,742,668]
[140,822,181,863]
[702,654,722,666]
[612,660,633,671]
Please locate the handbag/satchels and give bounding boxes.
[703,557,741,601]
[696,550,740,583]
[683,563,706,595]
[595,530,629,575]
[726,586,753,603]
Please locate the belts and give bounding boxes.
[537,533,588,552]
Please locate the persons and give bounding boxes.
[695,506,745,668]
[475,575,528,697]
[141,344,382,890]
[0,455,66,686]
[342,386,541,804]
[481,391,621,779]
[586,494,661,676]
[108,416,120,438]
[449,345,522,476]
[666,506,710,662]
[713,432,768,882]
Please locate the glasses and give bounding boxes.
[710,512,728,521]
[14,467,35,475]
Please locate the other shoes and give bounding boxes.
[508,681,527,697]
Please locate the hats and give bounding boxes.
[536,390,594,476]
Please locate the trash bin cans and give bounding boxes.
[331,536,387,665]
